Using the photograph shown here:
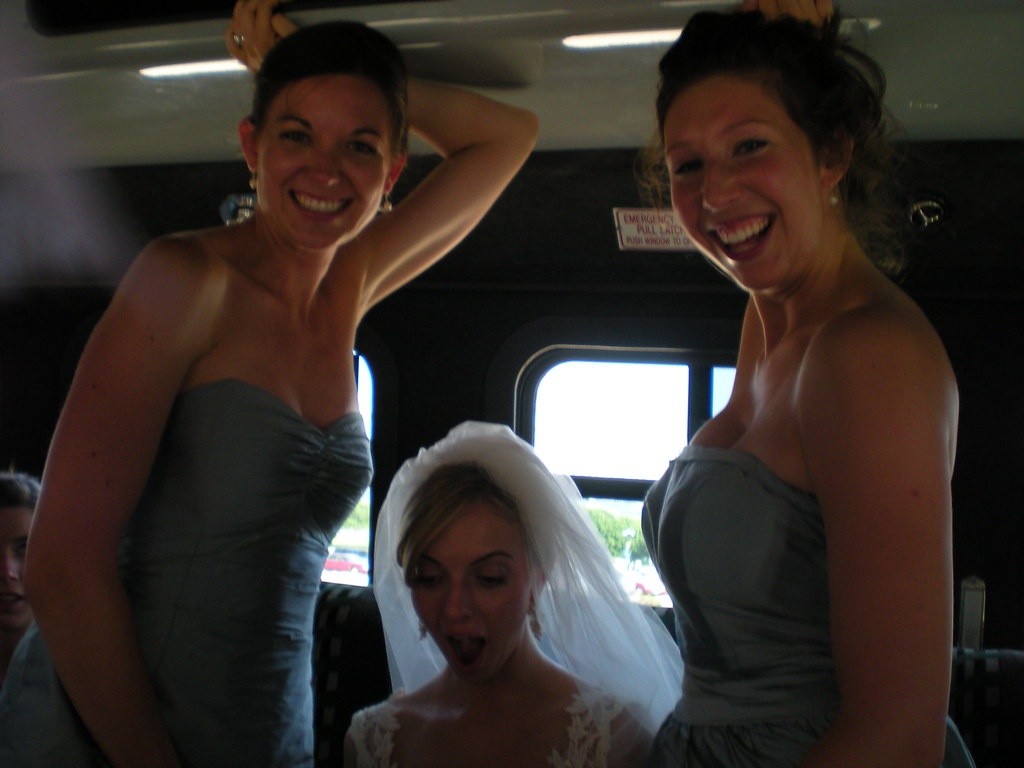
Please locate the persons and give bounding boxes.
[0,472,41,684]
[640,0,979,768]
[0,0,539,768]
[346,422,654,768]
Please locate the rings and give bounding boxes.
[232,33,246,47]
